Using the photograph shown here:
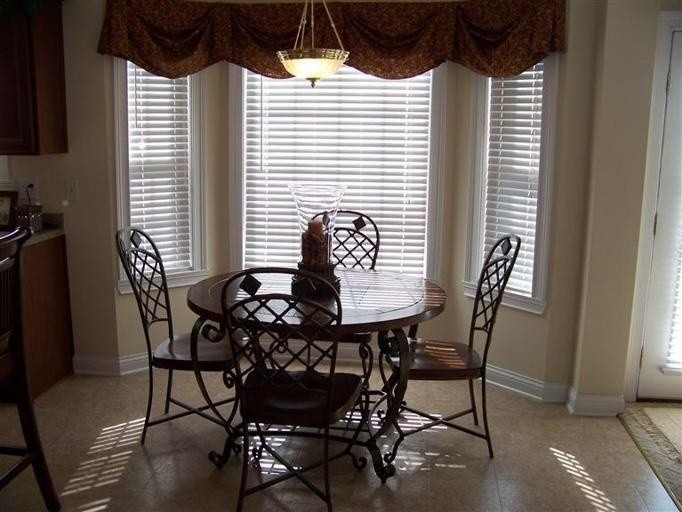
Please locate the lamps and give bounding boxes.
[276,1,351,89]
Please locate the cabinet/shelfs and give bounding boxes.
[0,0,68,156]
[20,234,74,404]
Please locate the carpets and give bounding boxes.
[618,402,682,512]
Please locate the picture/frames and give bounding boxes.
[0,190,19,232]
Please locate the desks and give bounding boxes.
[187,269,447,484]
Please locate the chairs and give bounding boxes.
[220,267,362,512]
[116,227,250,454]
[309,210,380,270]
[1,225,62,512]
[383,234,521,464]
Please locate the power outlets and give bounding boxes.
[18,178,40,201]
